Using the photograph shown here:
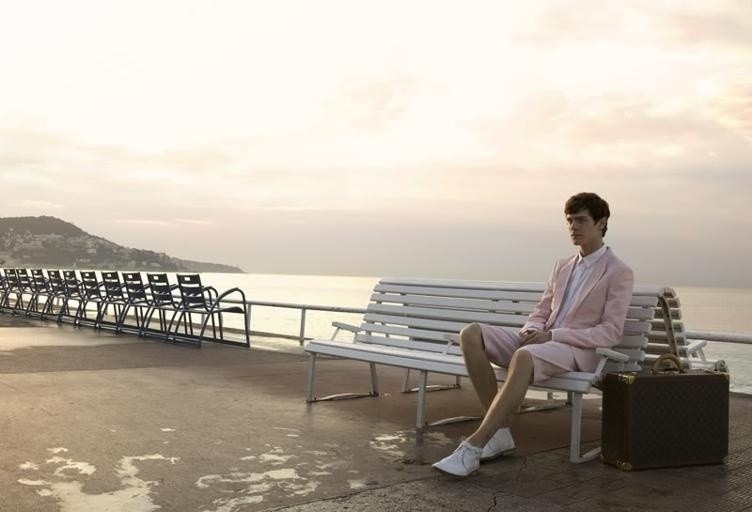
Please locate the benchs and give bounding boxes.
[300,277,711,465]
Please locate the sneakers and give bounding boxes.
[429,437,484,479]
[480,424,517,464]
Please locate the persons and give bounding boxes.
[432,192,635,480]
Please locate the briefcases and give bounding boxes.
[595,354,729,473]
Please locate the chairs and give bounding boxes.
[0,265,254,351]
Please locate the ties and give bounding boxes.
[551,264,589,331]
